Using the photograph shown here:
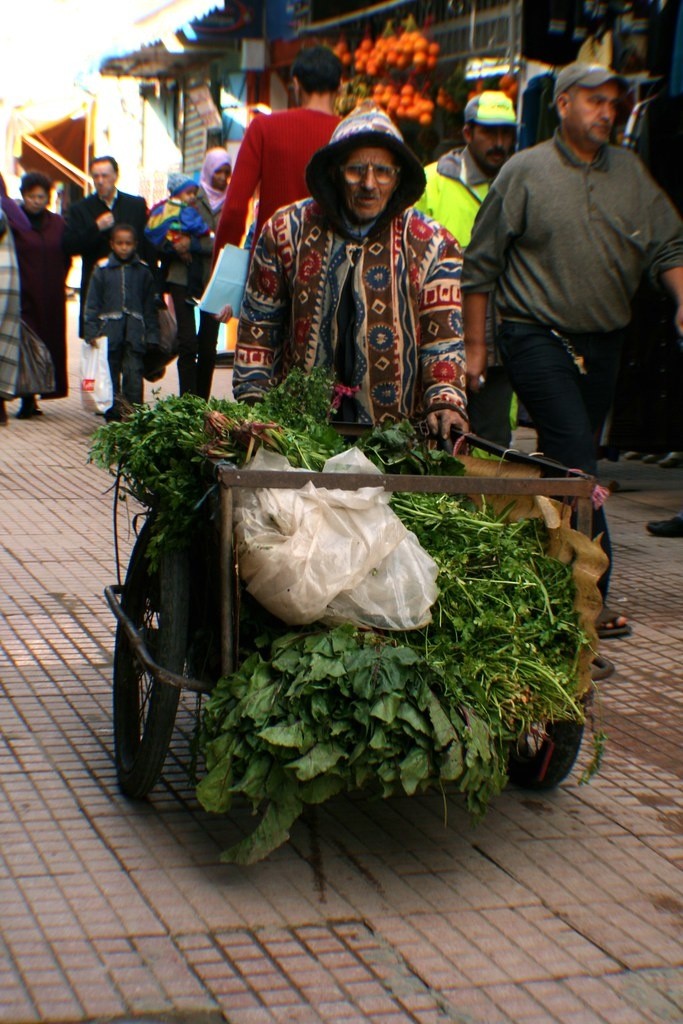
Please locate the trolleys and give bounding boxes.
[102,408,609,807]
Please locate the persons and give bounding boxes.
[210,45,346,324]
[0,173,55,424]
[166,147,233,402]
[84,225,162,419]
[145,173,215,315]
[414,91,519,431]
[232,111,482,438]
[462,60,683,637]
[20,174,68,398]
[66,156,147,338]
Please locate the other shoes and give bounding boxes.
[646,517,683,537]
[34,398,43,415]
[623,450,645,460]
[657,451,683,468]
[642,453,663,463]
[17,403,33,418]
[153,292,169,310]
[181,295,198,306]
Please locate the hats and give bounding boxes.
[167,172,199,197]
[554,62,630,102]
[464,90,519,127]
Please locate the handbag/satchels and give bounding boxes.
[81,336,114,415]
[17,320,55,395]
[141,298,178,377]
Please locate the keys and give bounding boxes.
[574,357,588,375]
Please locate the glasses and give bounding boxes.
[338,163,401,185]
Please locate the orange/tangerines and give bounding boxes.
[327,28,518,127]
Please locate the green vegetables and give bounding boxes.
[81,361,606,869]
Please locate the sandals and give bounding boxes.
[594,607,632,636]
